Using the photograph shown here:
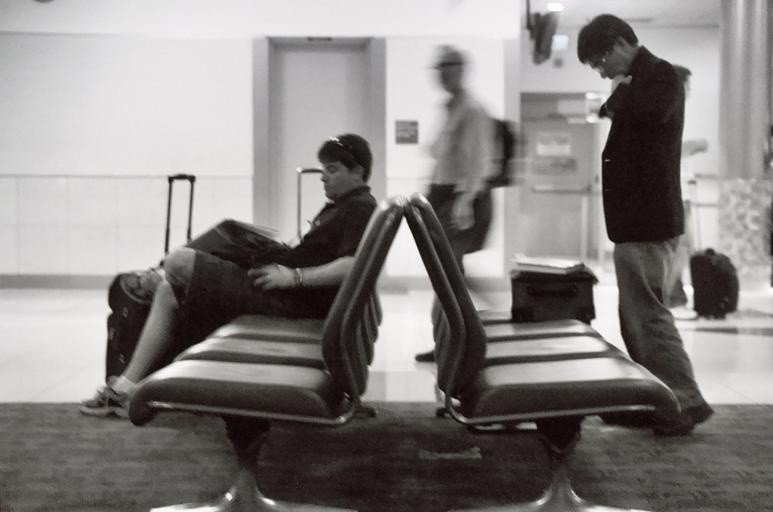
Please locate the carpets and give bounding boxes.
[0,401,772,512]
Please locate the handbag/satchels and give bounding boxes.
[184,219,292,267]
[495,118,514,189]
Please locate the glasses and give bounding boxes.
[591,49,609,70]
[328,135,344,148]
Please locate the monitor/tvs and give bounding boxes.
[527,9,561,66]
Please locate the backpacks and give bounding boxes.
[690,248,737,317]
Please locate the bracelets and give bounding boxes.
[294,267,303,287]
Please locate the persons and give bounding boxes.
[414,42,519,363]
[576,14,716,437]
[79,133,379,419]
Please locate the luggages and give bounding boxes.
[509,264,596,322]
[106,174,196,383]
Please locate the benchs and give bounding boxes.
[127,199,406,512]
[406,192,678,512]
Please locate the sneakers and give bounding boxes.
[80,388,131,418]
[651,404,712,436]
